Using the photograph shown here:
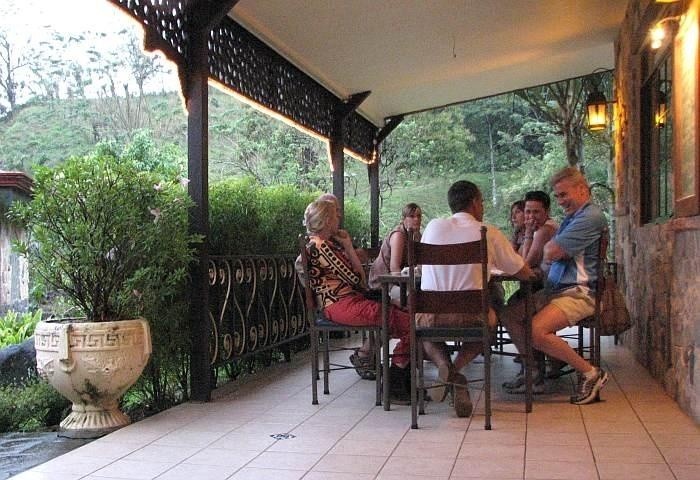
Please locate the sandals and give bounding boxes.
[349,349,376,380]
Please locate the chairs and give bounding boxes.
[407,226,491,430]
[532,228,604,402]
[299,234,380,406]
[361,238,400,345]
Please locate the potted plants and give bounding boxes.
[5,150,207,440]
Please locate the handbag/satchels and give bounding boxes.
[599,256,632,336]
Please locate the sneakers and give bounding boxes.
[429,361,456,403]
[570,365,611,405]
[450,372,474,417]
[502,373,546,394]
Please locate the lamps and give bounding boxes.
[582,67,618,132]
[651,15,684,49]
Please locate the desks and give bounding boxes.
[381,267,532,414]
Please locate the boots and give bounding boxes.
[382,364,411,406]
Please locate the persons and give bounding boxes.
[500,168,610,405]
[510,200,528,256]
[509,191,559,305]
[415,181,530,418]
[304,200,414,406]
[368,202,423,301]
[293,195,390,381]
